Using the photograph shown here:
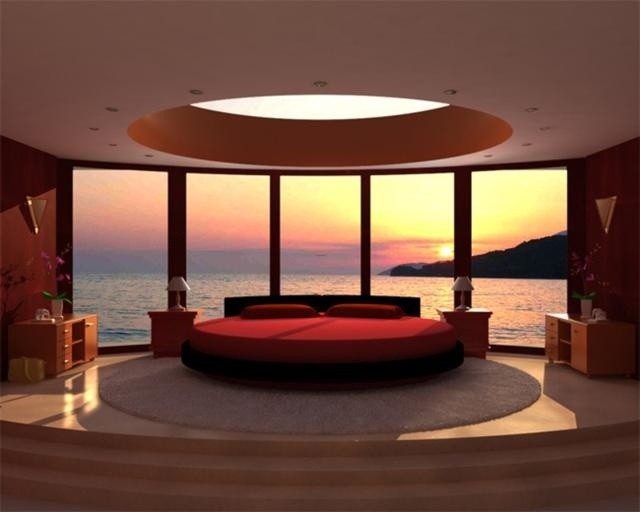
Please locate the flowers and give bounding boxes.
[567,241,610,300]
[36,246,76,303]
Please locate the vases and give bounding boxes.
[49,300,64,319]
[581,296,592,319]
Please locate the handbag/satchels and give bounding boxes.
[8,359,45,383]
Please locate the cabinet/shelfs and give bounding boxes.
[544,312,637,383]
[8,310,99,377]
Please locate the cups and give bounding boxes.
[35,307,51,321]
[595,311,608,320]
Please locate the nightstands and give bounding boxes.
[435,305,493,360]
[147,308,203,359]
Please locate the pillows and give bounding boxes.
[238,304,321,320]
[324,304,408,320]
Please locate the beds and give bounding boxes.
[181,291,465,394]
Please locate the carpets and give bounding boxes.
[96,353,542,439]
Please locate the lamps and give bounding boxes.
[163,276,189,310]
[26,195,48,233]
[450,275,474,310]
[595,195,618,232]
